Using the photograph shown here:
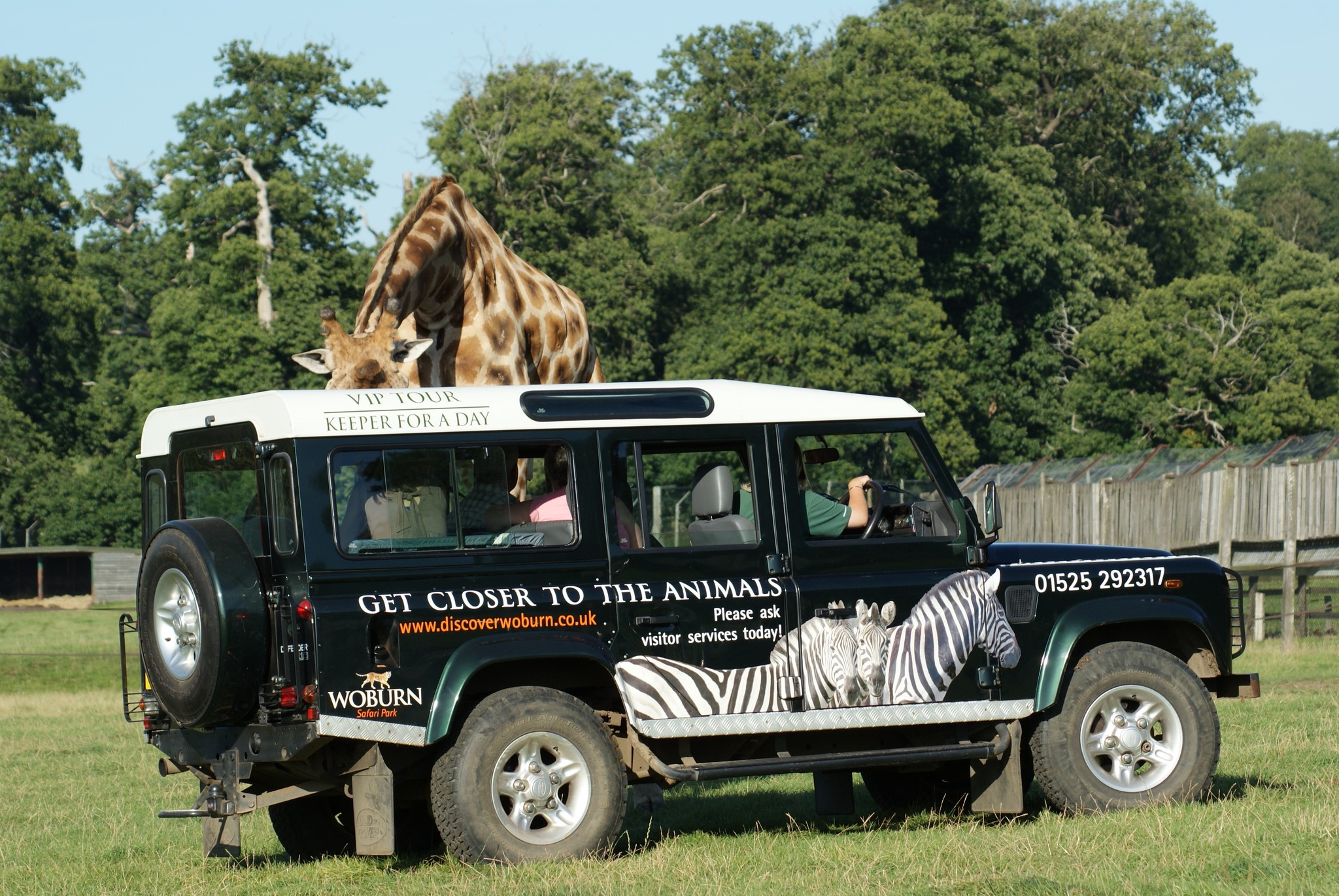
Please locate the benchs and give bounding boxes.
[348,531,544,555]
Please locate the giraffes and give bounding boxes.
[294,179,604,502]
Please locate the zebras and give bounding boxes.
[613,568,1020,722]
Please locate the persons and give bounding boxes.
[243,470,289,556]
[733,441,873,537]
[341,452,447,539]
[447,447,519,531]
[482,445,643,548]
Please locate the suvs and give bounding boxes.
[117,380,1262,876]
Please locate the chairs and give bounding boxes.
[509,520,574,546]
[688,463,757,545]
